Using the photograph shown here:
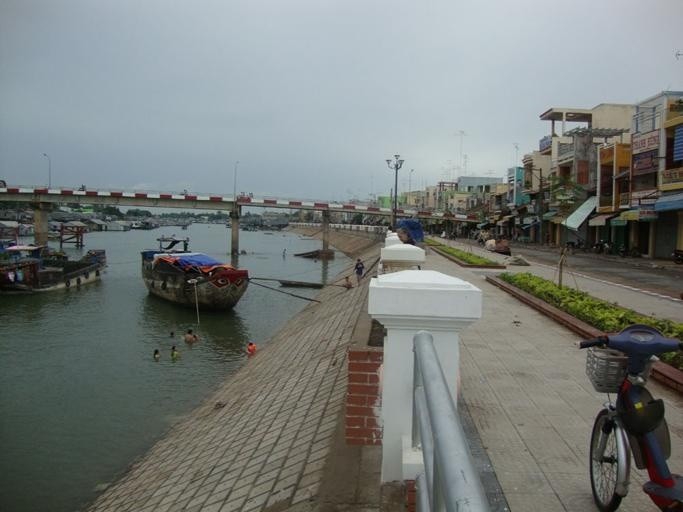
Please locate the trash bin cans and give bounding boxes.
[566,241,575,255]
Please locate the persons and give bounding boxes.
[343,276,353,289]
[246,342,256,353]
[154,349,160,359]
[170,332,174,337]
[184,329,193,344]
[193,334,198,341]
[170,346,178,359]
[352,258,365,286]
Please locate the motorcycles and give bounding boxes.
[566,238,683,264]
[581,325,683,512]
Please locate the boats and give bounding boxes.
[141,233,249,309]
[0,244,109,294]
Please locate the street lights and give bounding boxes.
[386,155,404,233]
[43,153,52,190]
[233,160,240,210]
[518,167,544,246]
